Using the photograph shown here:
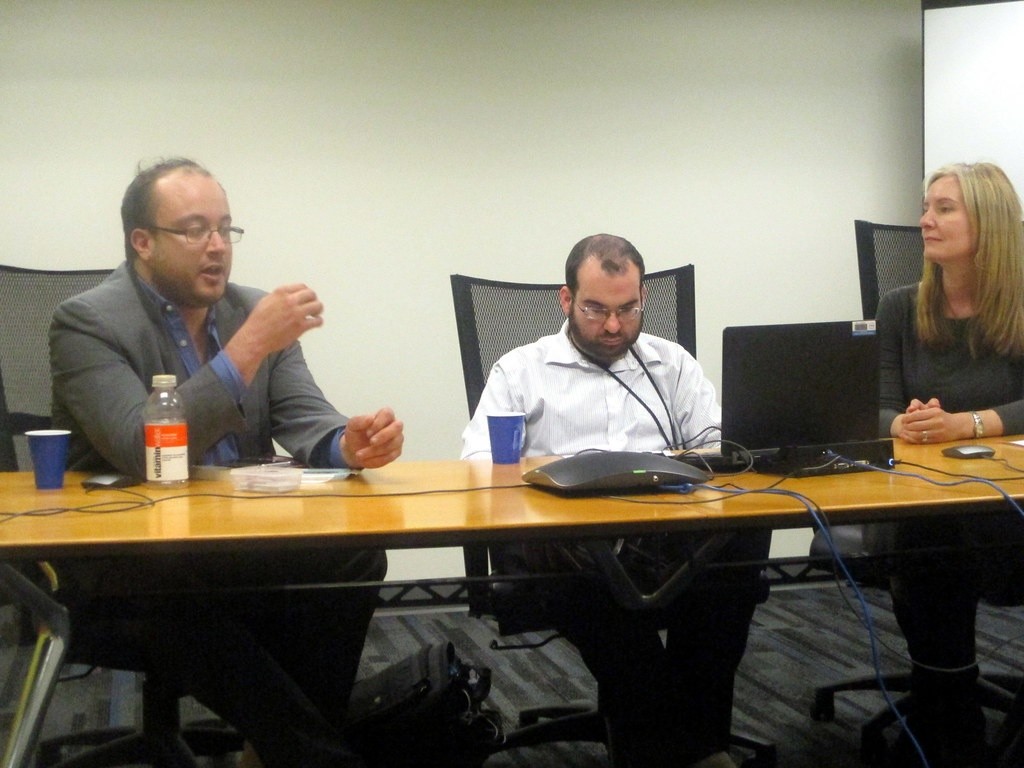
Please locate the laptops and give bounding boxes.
[668,320,881,473]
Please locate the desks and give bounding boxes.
[0,423,1024,768]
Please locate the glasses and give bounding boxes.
[138,222,245,245]
[569,288,646,320]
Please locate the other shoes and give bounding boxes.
[692,750,738,768]
[879,707,986,768]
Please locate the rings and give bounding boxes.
[923,431,927,442]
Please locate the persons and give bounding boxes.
[460,233,758,768]
[49,159,404,768]
[876,164,1024,768]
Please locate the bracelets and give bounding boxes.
[970,411,983,439]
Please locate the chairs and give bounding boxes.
[0,265,296,768]
[809,218,1023,768]
[446,260,781,767]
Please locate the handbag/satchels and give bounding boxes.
[344,640,508,768]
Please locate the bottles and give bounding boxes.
[143,374,190,489]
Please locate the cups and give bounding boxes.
[487,411,525,465]
[23,430,71,490]
